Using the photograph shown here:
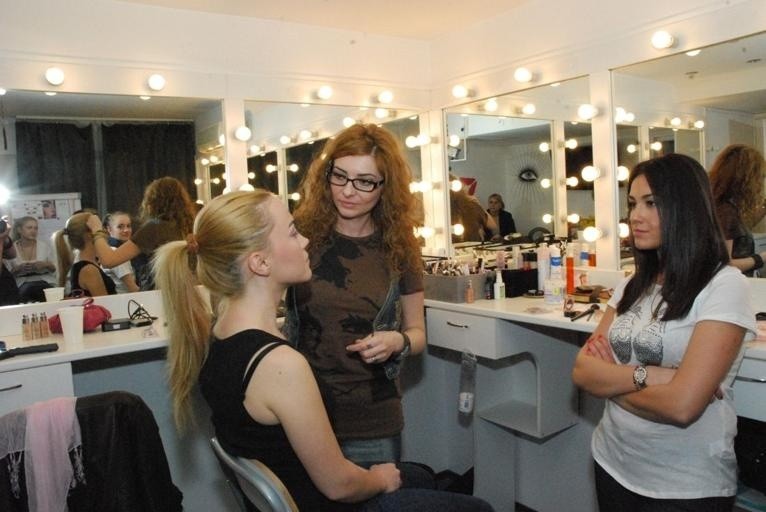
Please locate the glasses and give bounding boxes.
[327,171,386,193]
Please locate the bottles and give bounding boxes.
[535,242,562,292]
[21,312,49,338]
[580,251,596,267]
[493,243,530,272]
[485,269,507,300]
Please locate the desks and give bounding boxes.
[399,283,766,510]
[0,307,243,512]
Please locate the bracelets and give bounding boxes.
[750,254,764,270]
[395,331,411,363]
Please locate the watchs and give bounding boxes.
[634,363,648,389]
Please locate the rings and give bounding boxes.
[372,357,377,362]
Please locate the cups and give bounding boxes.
[545,280,564,309]
[58,306,85,341]
[42,287,64,302]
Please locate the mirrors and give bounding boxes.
[617,120,705,270]
[443,74,597,273]
[446,110,596,255]
[204,160,226,206]
[1,88,227,316]
[244,98,426,278]
[247,115,426,252]
[610,32,766,283]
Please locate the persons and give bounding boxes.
[708,142,766,279]
[449,171,496,242]
[276,125,430,485]
[483,192,518,244]
[0,176,202,308]
[572,150,758,510]
[146,187,495,511]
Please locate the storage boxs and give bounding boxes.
[418,262,491,302]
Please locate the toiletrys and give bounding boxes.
[19,311,50,340]
[484,271,494,298]
[537,242,552,292]
[493,270,506,300]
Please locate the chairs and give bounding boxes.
[209,436,303,511]
[0,390,187,512]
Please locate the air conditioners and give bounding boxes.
[194,121,224,155]
